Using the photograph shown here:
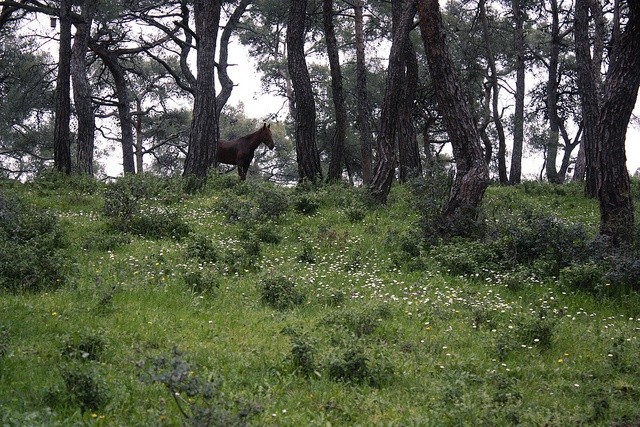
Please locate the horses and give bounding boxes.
[216,122,275,181]
